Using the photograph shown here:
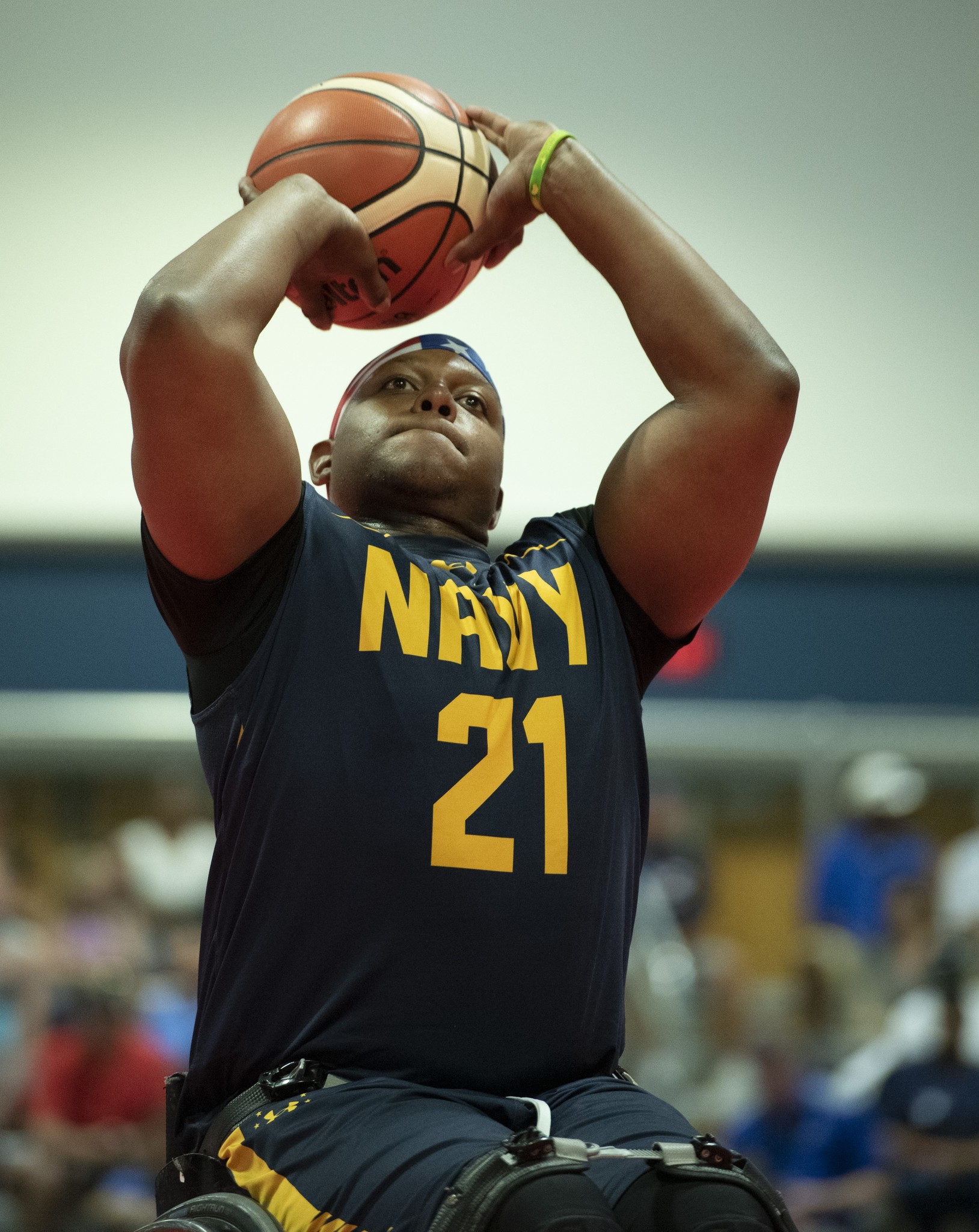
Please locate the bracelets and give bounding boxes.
[529,129,574,214]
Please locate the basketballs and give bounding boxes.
[246,73,499,332]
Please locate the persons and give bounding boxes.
[0,752,979,1232]
[116,107,801,1232]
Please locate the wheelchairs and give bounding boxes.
[132,1074,288,1231]
